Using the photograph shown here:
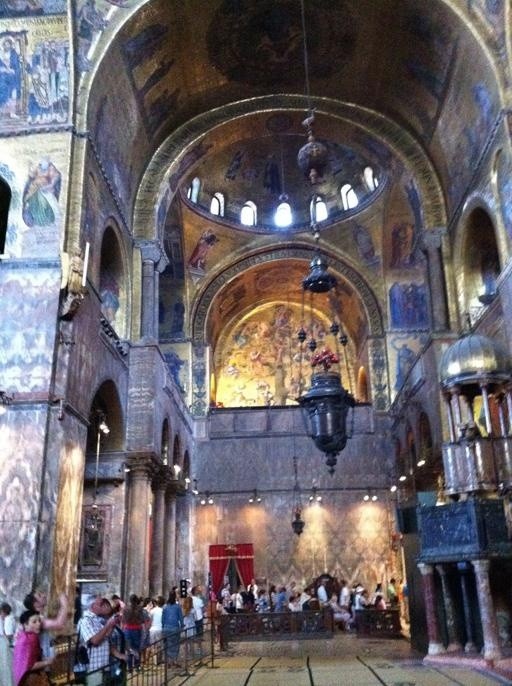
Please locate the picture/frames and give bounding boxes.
[76,504,112,583]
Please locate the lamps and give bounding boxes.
[249,489,261,503]
[201,491,214,505]
[363,487,378,502]
[99,410,110,435]
[309,486,322,502]
[192,479,198,495]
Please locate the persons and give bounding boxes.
[0,584,199,681]
[202,578,408,634]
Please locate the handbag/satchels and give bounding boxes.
[73,646,89,683]
